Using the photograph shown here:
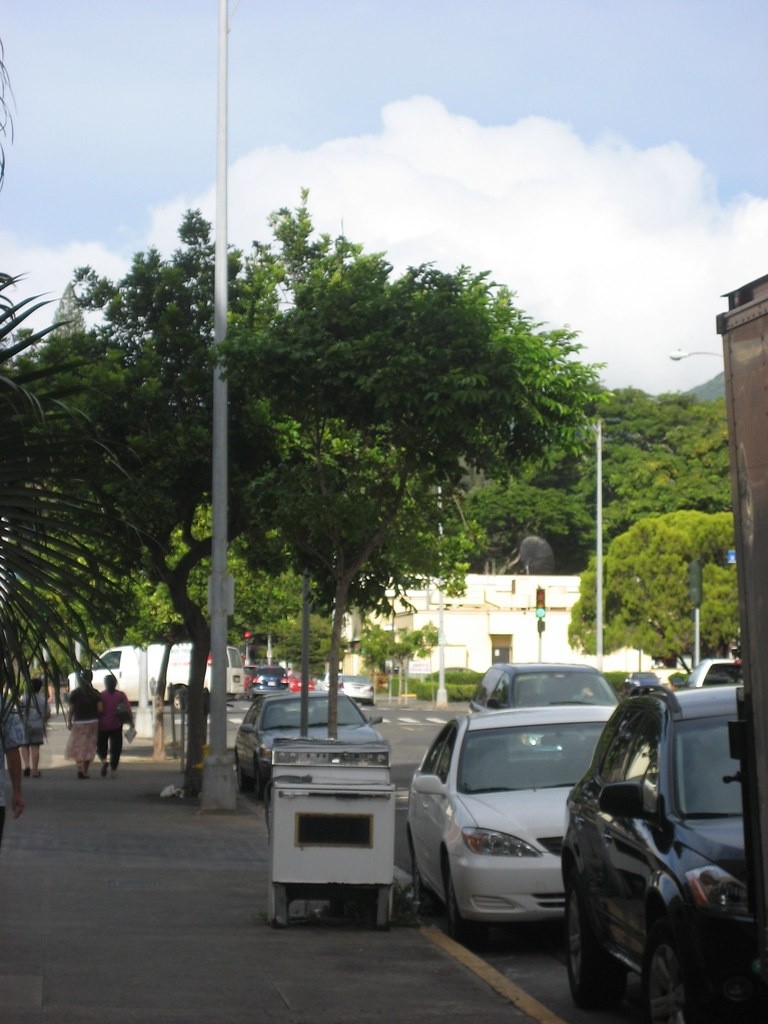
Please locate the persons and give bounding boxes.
[17,679,51,777]
[64,670,104,777]
[0,690,26,846]
[97,675,135,777]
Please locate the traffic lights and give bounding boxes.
[683,561,704,606]
[536,587,546,618]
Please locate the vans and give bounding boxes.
[467,661,625,769]
[672,659,743,693]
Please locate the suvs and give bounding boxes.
[559,683,752,1024]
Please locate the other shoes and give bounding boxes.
[84,772,91,778]
[110,770,117,779]
[77,771,83,778]
[33,771,41,778]
[23,766,31,777]
[100,759,109,778]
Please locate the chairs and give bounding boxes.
[476,737,509,784]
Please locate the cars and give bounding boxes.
[625,671,658,691]
[243,665,257,692]
[314,673,375,708]
[235,690,391,800]
[286,671,315,693]
[405,704,629,940]
[247,665,289,701]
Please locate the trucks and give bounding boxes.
[67,641,244,711]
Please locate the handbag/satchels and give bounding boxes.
[115,692,131,723]
